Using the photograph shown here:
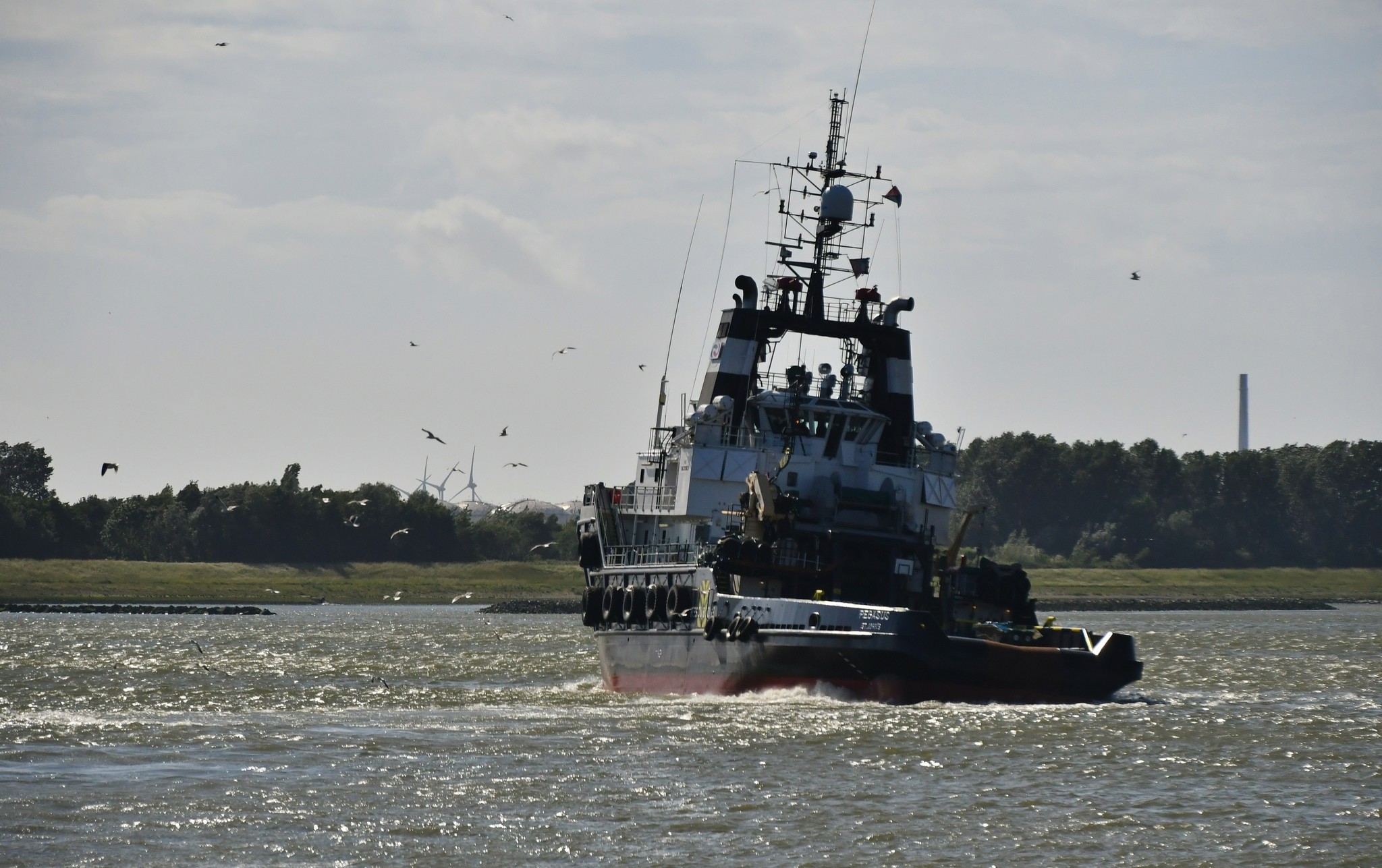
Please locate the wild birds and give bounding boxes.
[639,364,647,371]
[498,425,509,437]
[450,590,475,604]
[530,542,559,551]
[421,428,446,444]
[347,499,374,505]
[189,639,203,653]
[342,514,363,527]
[552,347,577,359]
[216,494,238,513]
[1131,270,1141,280]
[502,462,529,468]
[371,675,389,690]
[410,341,420,346]
[314,497,330,502]
[215,42,229,46]
[447,467,466,474]
[101,462,119,476]
[390,528,410,539]
[384,590,405,601]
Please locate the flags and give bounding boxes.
[881,185,902,208]
[850,257,870,279]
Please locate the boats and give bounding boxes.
[573,85,1145,708]
